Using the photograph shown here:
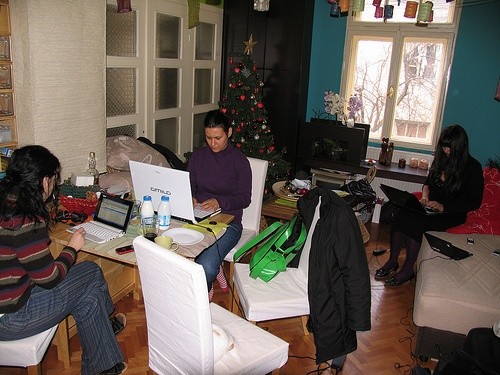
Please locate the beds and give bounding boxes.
[412,231,500,362]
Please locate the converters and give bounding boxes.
[331,354,347,372]
[373,248,387,256]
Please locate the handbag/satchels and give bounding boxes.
[233,215,307,282]
[338,166,376,208]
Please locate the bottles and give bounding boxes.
[157,195,171,231]
[384,142,394,165]
[87,152,100,185]
[141,195,155,228]
[379,137,389,164]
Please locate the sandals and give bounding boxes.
[98,362,127,375]
[109,313,127,336]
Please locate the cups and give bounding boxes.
[0,35,10,61]
[419,159,428,169]
[410,156,418,168]
[142,217,160,242]
[154,236,178,252]
[0,92,13,115]
[399,156,407,168]
[0,64,11,88]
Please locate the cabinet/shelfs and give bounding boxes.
[0,0,18,174]
[221,0,315,162]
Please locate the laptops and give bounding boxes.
[424,233,474,261]
[128,159,222,225]
[380,183,448,215]
[65,193,135,243]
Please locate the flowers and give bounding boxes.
[324,89,363,125]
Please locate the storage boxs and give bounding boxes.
[71,171,94,187]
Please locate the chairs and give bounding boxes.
[232,196,321,335]
[133,236,289,375]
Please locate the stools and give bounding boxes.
[0,314,58,375]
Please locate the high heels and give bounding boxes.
[385,271,414,286]
[375,262,399,279]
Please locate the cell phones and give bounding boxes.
[467,237,474,245]
[115,245,134,255]
[492,248,500,257]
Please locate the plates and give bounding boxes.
[272,181,299,202]
[162,228,204,246]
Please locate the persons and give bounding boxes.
[183,110,253,303]
[374,124,485,286]
[0,144,127,375]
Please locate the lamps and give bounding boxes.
[253,0,269,11]
[116,0,132,13]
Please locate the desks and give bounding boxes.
[49,205,234,369]
[312,174,346,193]
[355,158,429,184]
[262,201,297,220]
